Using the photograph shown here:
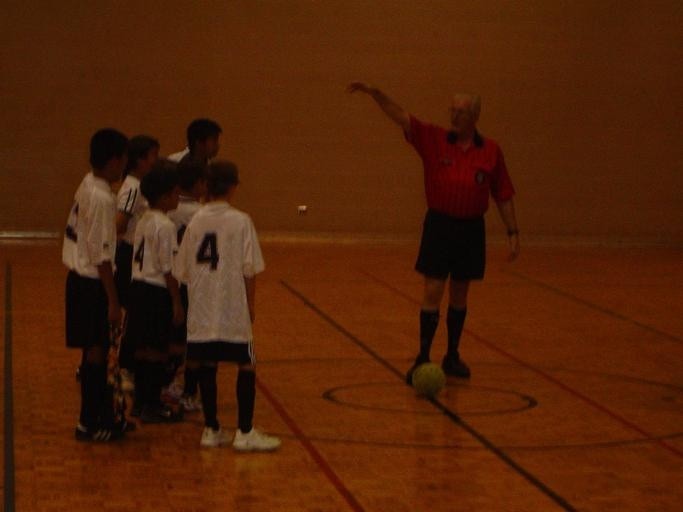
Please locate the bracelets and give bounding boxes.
[506,227,518,237]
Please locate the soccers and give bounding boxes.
[413,364,445,395]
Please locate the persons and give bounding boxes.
[171,160,280,450]
[345,79,521,387]
[62,118,222,443]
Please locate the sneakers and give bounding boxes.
[406,354,431,384]
[442,352,470,378]
[75,379,201,444]
[234,428,282,450]
[200,426,235,447]
